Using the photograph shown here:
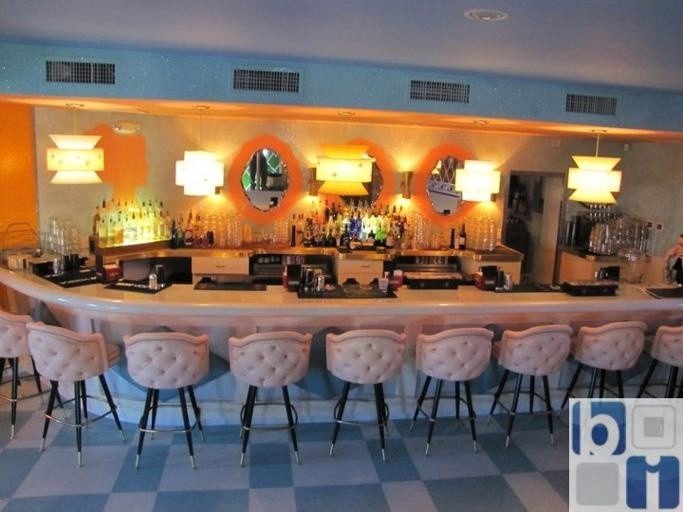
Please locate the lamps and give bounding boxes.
[43,103,104,185]
[172,104,228,199]
[313,111,376,199]
[451,120,500,204]
[564,129,624,208]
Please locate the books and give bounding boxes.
[298,281,399,299]
[645,284,682,299]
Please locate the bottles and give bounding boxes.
[287,199,411,252]
[449,223,466,250]
[92,193,203,249]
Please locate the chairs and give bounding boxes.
[0,310,682,468]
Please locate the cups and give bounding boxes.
[393,270,403,288]
[208,213,287,247]
[378,277,390,293]
[496,269,504,290]
[303,268,326,290]
[410,210,441,251]
[154,265,165,287]
[589,213,646,262]
[504,273,512,290]
[462,215,497,252]
[148,274,157,290]
[43,215,77,273]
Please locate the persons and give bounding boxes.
[662,233,683,283]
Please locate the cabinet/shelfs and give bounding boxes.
[556,251,624,288]
[457,254,523,285]
[331,258,386,286]
[186,257,249,287]
